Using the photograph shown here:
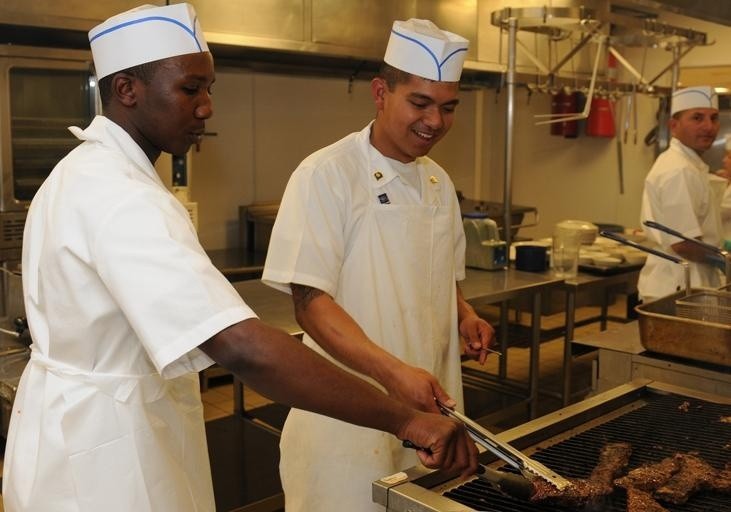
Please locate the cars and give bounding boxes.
[551,229,580,279]
[516,245,550,273]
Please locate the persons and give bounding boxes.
[636,85,731,304]
[261,16,495,511]
[1,3,479,512]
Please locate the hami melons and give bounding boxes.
[527,443,730,512]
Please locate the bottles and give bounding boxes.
[383,18,470,83]
[670,86,719,118]
[87,2,210,83]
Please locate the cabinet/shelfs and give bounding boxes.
[553,94,615,138]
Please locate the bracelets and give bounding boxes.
[552,228,648,268]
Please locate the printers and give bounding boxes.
[401,400,573,507]
[623,92,639,145]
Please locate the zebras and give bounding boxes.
[0,43,197,245]
